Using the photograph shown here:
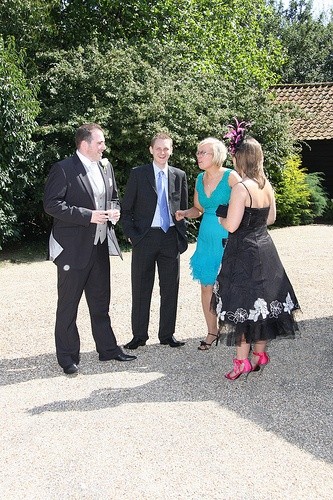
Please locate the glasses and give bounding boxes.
[195,150,213,156]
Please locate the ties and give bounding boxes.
[157,171,170,233]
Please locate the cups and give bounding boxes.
[107,201,118,219]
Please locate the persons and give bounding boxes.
[118,130,189,349]
[209,115,304,381]
[43,124,137,374]
[174,138,242,350]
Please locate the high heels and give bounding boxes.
[249,351,269,380]
[197,332,220,350]
[225,358,253,382]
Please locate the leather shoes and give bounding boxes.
[64,362,78,374]
[123,338,146,350]
[99,352,138,361]
[160,335,185,348]
